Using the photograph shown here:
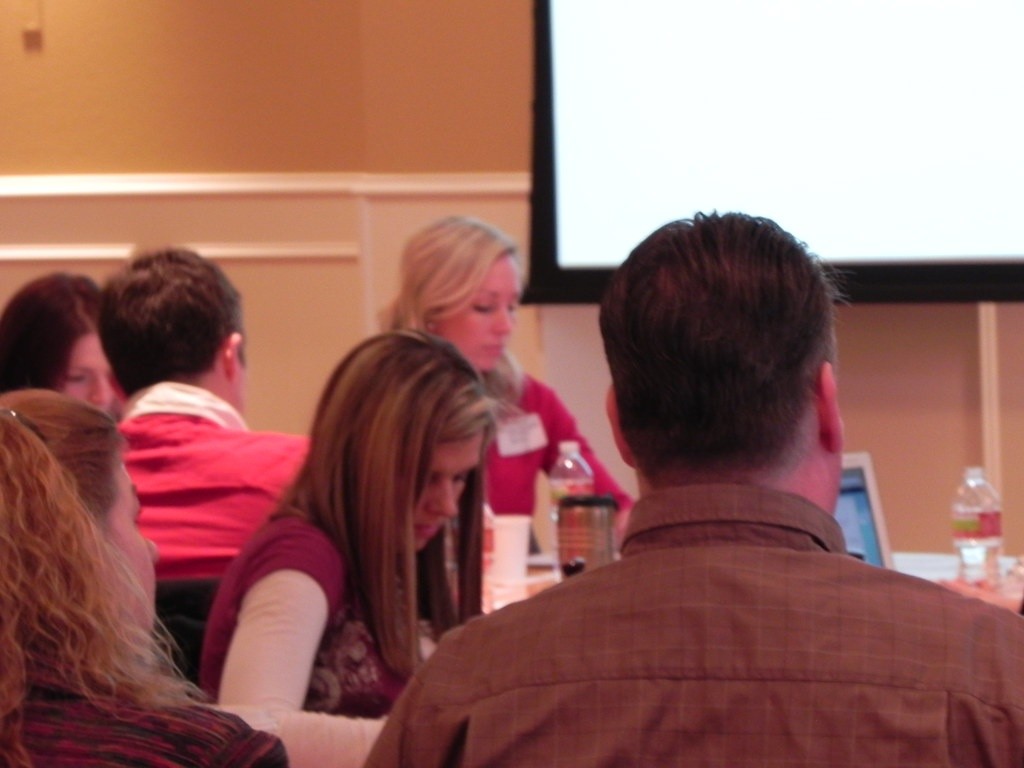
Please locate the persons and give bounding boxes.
[2,388,287,767]
[358,210,1023,767]
[1,273,123,423]
[380,215,637,557]
[95,249,311,581]
[200,329,498,718]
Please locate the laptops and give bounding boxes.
[827,450,892,585]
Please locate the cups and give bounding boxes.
[556,495,622,584]
[482,514,532,584]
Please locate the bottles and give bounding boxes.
[951,466,1004,592]
[548,441,598,585]
